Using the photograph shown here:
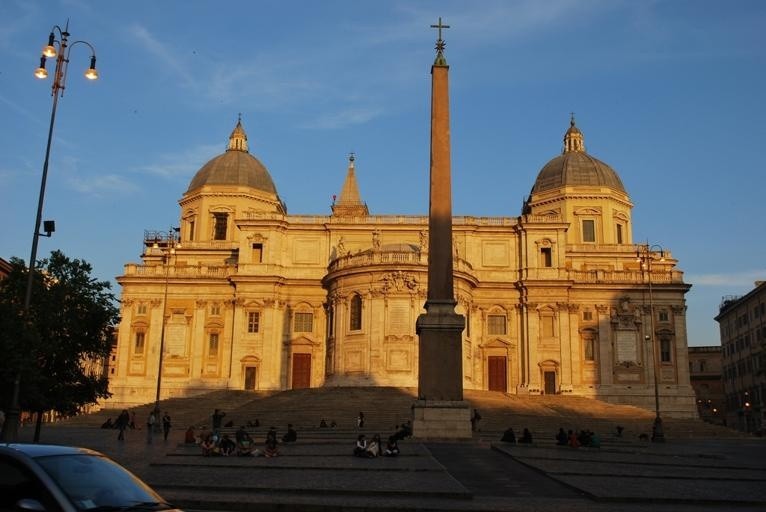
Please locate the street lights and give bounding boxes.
[636,238,668,445]
[144,223,183,432]
[1,17,99,443]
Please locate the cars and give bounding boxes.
[0,443,185,512]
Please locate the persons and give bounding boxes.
[518,428,532,443]
[556,428,599,449]
[473,409,481,432]
[501,428,516,443]
